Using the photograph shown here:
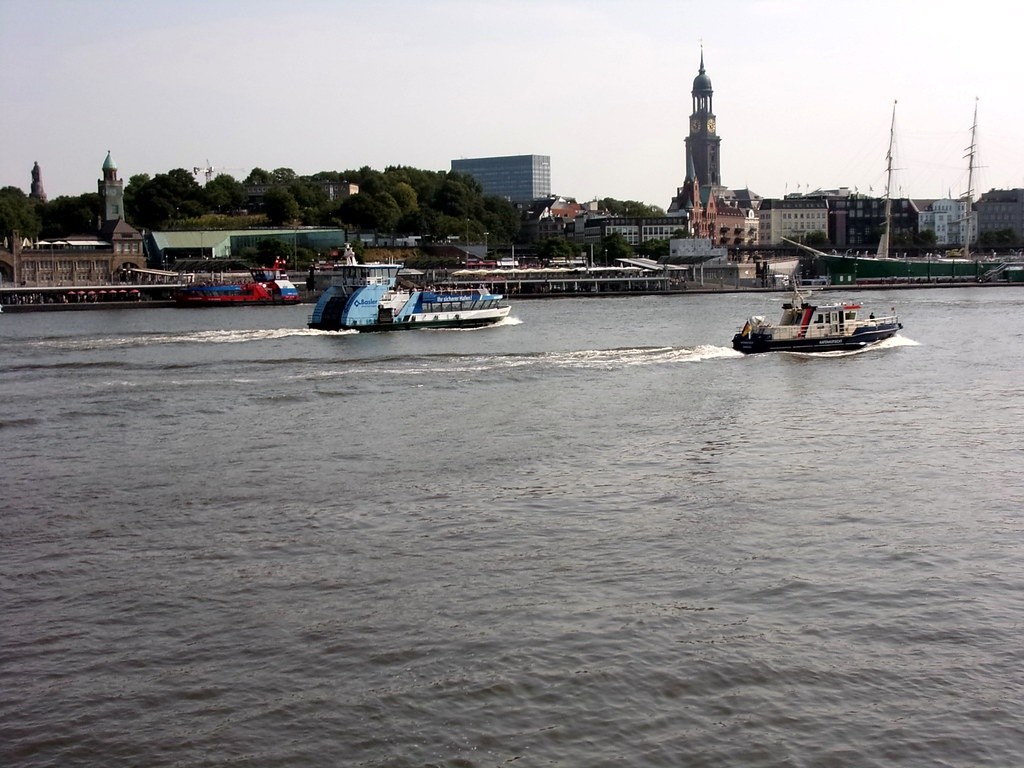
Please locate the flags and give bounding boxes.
[742,320,750,337]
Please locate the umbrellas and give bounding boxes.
[66,289,140,295]
[454,265,644,281]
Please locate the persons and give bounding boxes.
[11,291,98,303]
[669,276,688,288]
[396,283,471,297]
[869,312,875,319]
[187,278,252,287]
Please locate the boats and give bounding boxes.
[728,273,904,355]
[175,267,302,308]
[306,241,513,333]
